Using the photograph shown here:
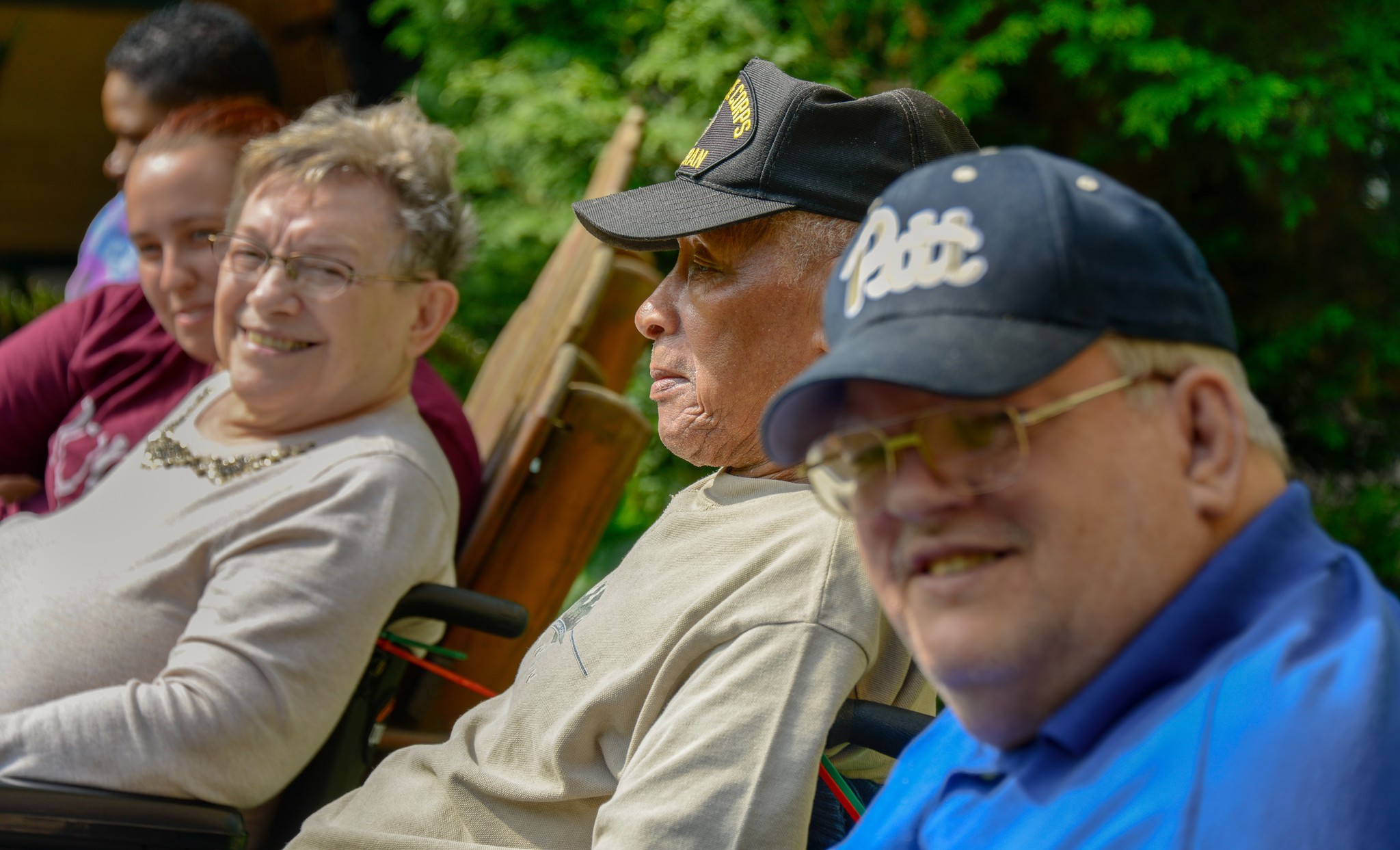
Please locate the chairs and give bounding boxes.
[383,105,661,747]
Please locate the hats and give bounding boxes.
[571,59,981,254]
[759,145,1241,470]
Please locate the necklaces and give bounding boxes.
[141,375,317,487]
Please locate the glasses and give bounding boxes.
[803,369,1176,520]
[208,231,435,299]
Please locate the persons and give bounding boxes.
[64,0,276,306]
[757,146,1400,850]
[0,88,479,818]
[279,59,982,850]
[0,97,487,565]
